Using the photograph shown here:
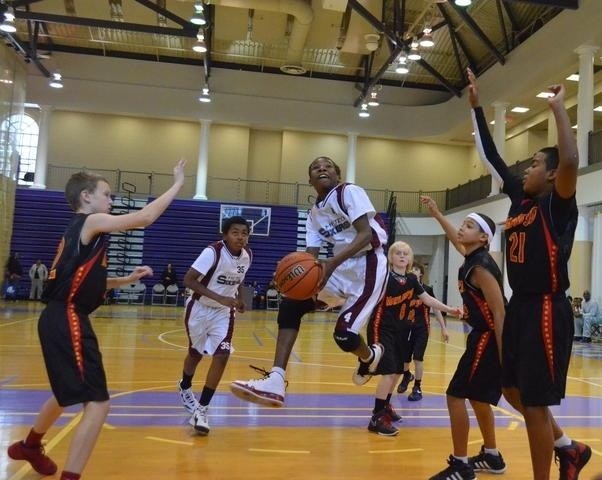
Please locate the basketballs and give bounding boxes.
[276,252,322,301]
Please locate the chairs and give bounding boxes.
[117,283,131,305]
[184,287,194,305]
[591,316,602,338]
[165,285,178,306]
[266,289,279,310]
[130,283,146,305]
[151,284,164,305]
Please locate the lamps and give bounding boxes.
[191,1,206,25]
[420,23,434,47]
[192,28,207,53]
[48,72,65,88]
[368,87,380,107]
[455,0,472,7]
[407,35,421,61]
[395,49,409,73]
[0,6,16,34]
[365,32,381,52]
[198,84,211,103]
[358,97,370,118]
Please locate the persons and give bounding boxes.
[250,281,261,308]
[397,260,449,400]
[8,251,23,302]
[465,65,593,480]
[28,259,48,300]
[160,263,177,288]
[567,289,602,343]
[418,193,508,480]
[362,239,463,436]
[173,216,253,436]
[228,155,391,408]
[6,159,187,480]
[265,280,275,298]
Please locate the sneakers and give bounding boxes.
[580,337,591,343]
[370,403,403,424]
[468,445,507,475]
[574,337,582,341]
[8,440,57,476]
[367,414,399,436]
[189,402,210,434]
[397,374,414,393]
[231,364,289,406]
[428,454,477,480]
[554,441,591,480]
[352,342,385,385]
[408,385,422,401]
[177,379,198,414]
